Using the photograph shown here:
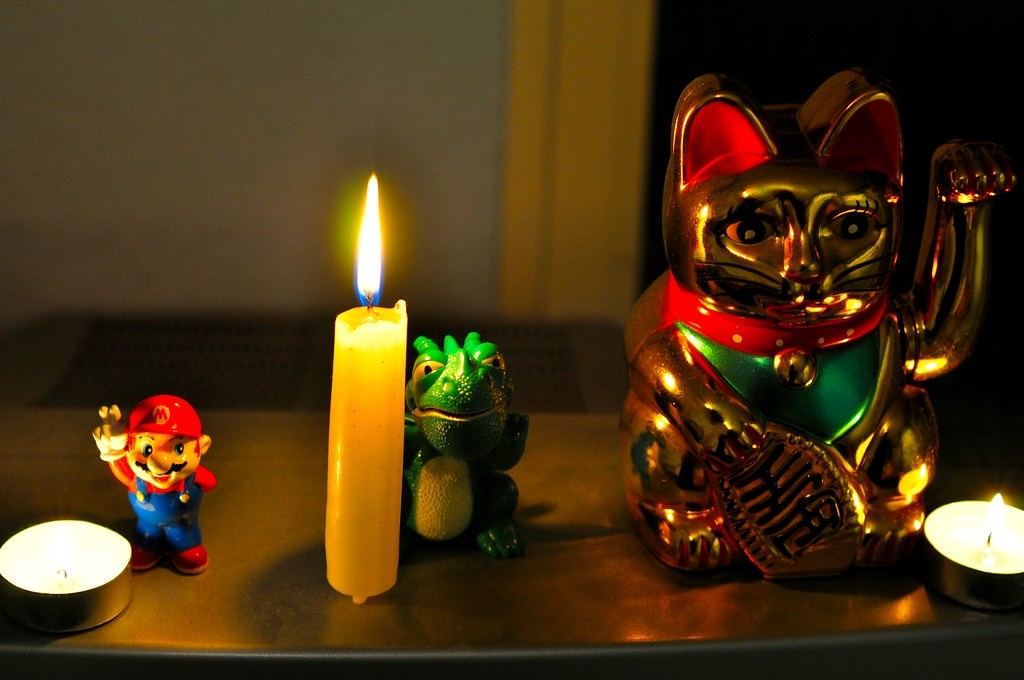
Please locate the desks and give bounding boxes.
[0,403,1024,680]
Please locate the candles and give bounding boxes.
[0,519,133,634]
[326,171,408,603]
[924,492,1024,611]
